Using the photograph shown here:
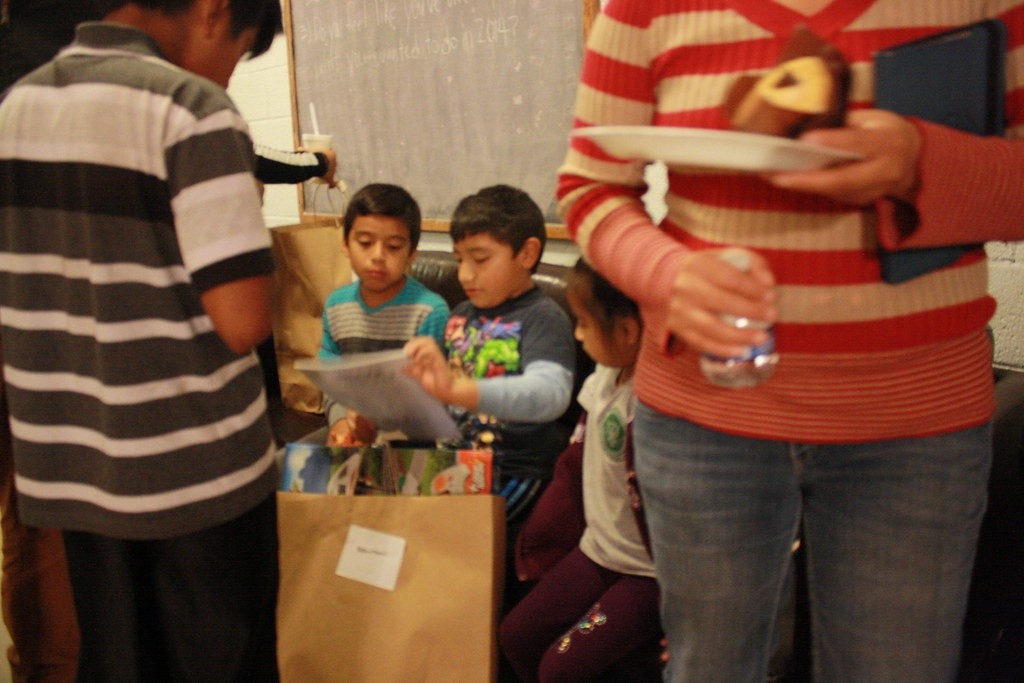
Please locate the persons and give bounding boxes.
[398,184,576,525]
[251,143,338,206]
[316,184,444,450]
[496,0,1024,683]
[0,0,286,683]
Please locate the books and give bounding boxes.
[293,347,463,445]
[335,522,409,596]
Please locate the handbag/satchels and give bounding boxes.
[870,17,1006,285]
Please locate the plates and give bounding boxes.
[572,124,865,173]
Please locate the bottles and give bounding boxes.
[700,249,777,388]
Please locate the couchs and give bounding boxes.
[253,250,586,683]
[955,328,1024,683]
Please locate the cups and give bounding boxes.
[302,133,334,184]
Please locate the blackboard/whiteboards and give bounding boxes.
[283,0,602,239]
